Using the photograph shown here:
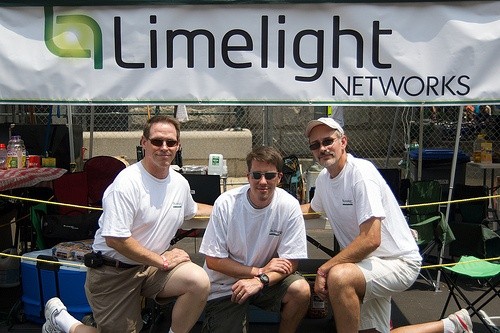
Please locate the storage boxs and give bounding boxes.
[21,246,94,324]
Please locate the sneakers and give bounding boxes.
[448,309,474,333]
[41,296,68,333]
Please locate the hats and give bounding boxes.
[304,117,345,138]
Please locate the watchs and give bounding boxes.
[256,274,269,289]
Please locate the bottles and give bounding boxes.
[473,134,487,161]
[6,135,26,170]
[0,144,7,170]
[480,140,492,165]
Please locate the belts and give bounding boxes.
[102,258,143,269]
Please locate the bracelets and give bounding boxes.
[317,270,326,278]
[160,255,169,271]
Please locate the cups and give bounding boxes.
[29,155,40,167]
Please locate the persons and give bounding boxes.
[42,114,211,333]
[199,146,310,333]
[300,117,473,333]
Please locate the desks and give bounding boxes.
[0,167,68,257]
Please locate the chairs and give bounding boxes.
[438,255,500,333]
[401,180,444,290]
[50,156,128,243]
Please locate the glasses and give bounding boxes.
[309,137,339,150]
[250,171,279,180]
[144,136,179,147]
